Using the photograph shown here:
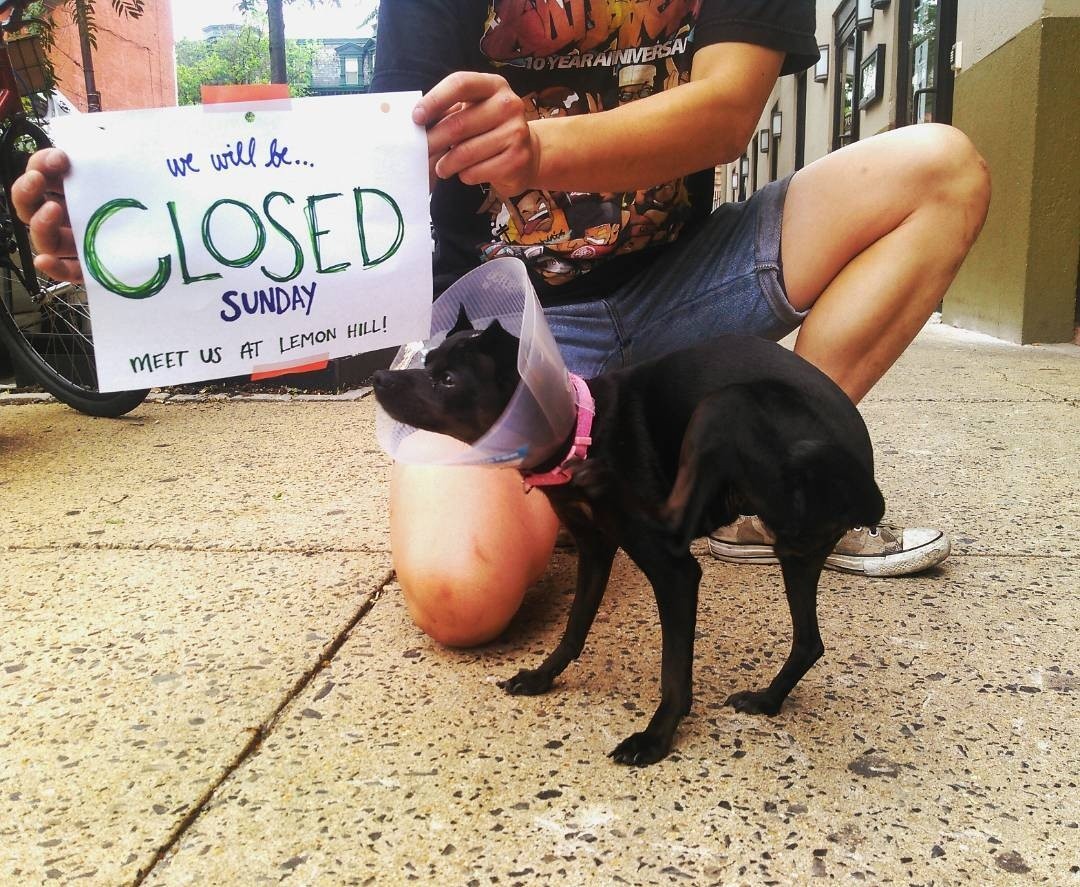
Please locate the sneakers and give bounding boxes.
[707,505,952,579]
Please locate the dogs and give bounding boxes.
[370,300,887,766]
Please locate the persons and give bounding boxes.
[10,0,994,648]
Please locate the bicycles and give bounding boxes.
[0,0,154,418]
